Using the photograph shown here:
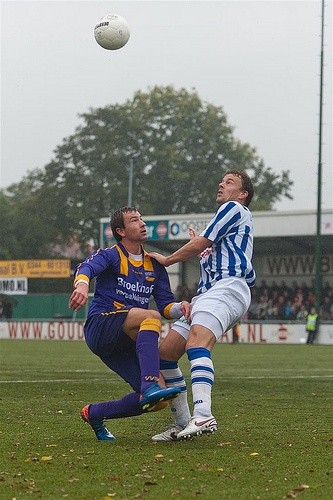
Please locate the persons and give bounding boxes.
[176,282,199,302]
[68,207,192,441]
[144,169,257,442]
[232,278,333,321]
[305,307,320,345]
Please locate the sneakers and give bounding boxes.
[177,416,217,439]
[139,384,181,412]
[152,420,194,442]
[81,404,115,440]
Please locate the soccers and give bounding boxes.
[94,14,130,50]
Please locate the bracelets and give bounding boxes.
[72,280,89,287]
